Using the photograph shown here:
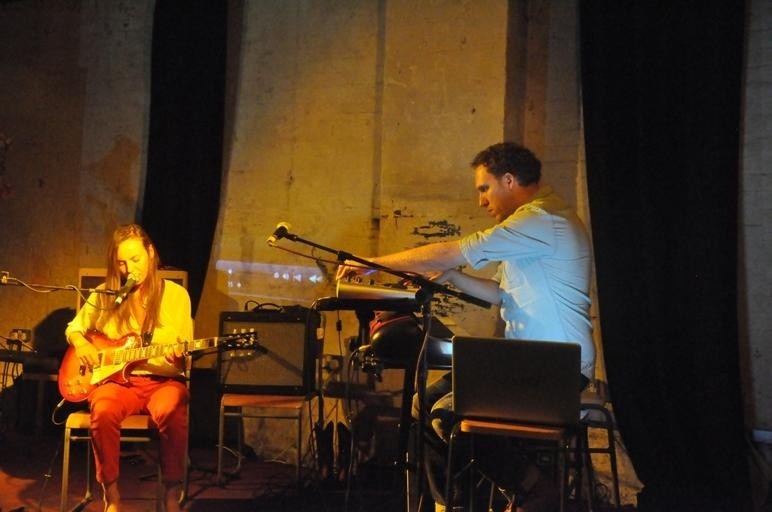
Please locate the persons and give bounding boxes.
[61,223,199,511]
[332,139,602,491]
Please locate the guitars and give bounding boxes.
[58,329,258,404]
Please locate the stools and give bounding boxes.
[441,419,590,512]
[579,387,621,512]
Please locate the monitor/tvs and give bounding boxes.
[214,308,323,397]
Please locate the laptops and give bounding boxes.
[450,335,583,429]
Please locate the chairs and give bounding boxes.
[216,308,321,493]
[59,269,191,512]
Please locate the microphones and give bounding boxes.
[115,272,139,305]
[267,221,292,244]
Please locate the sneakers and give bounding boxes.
[505,473,557,512]
